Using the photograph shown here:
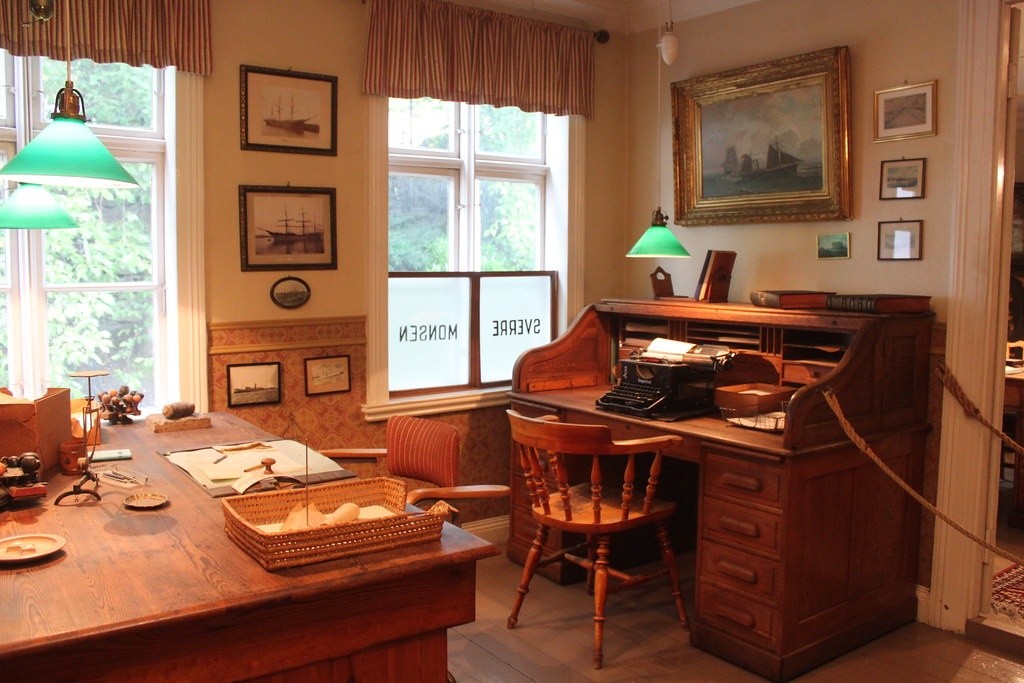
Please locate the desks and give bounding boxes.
[0,409,502,683]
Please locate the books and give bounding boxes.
[694,250,737,301]
[825,294,933,313]
[756,289,837,311]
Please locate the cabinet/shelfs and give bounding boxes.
[504,300,936,683]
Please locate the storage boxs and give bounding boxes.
[0,388,72,480]
[714,382,798,419]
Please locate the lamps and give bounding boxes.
[660,0,679,65]
[0,0,139,230]
[625,0,692,258]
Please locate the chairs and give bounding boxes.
[506,408,689,670]
[318,415,512,528]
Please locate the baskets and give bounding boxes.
[222,477,448,570]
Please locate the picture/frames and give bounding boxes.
[879,158,926,200]
[877,220,923,261]
[270,277,310,309]
[816,232,850,259]
[240,64,338,156]
[227,362,281,407]
[303,354,351,396]
[872,77,938,144]
[670,45,852,227]
[239,184,338,271]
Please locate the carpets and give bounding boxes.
[992,563,1024,618]
